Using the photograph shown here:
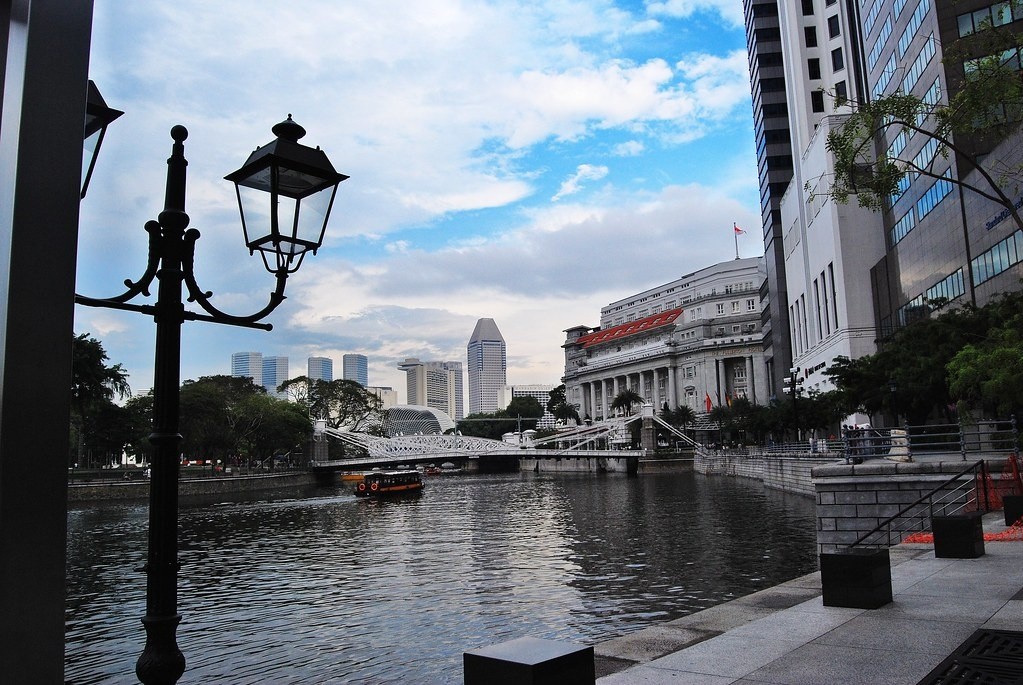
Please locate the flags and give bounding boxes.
[735,227,747,235]
[706,392,747,412]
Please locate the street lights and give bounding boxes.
[77,78,352,685]
[782,368,805,454]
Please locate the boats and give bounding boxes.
[351,467,427,498]
[423,464,442,476]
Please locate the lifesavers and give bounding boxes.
[359,484,366,490]
[371,483,378,490]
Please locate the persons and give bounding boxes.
[840,424,865,448]
